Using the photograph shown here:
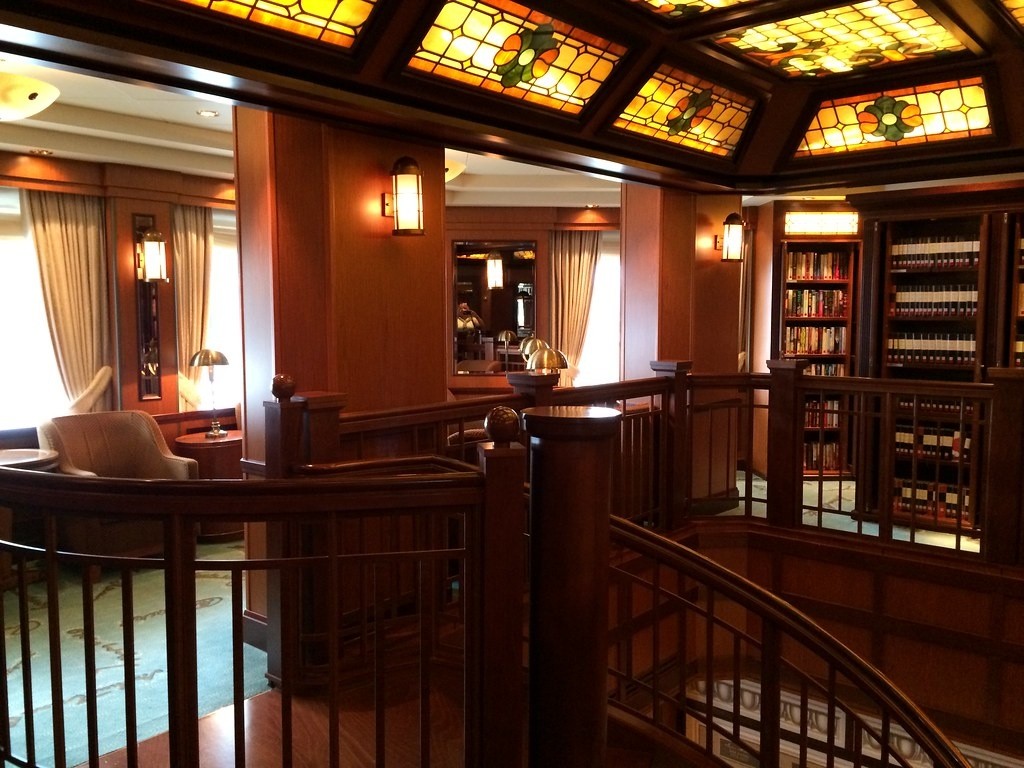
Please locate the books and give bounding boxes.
[1014,231,1024,367]
[785,231,981,522]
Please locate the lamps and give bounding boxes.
[715,212,744,263]
[189,349,229,439]
[445,158,467,183]
[0,72,61,122]
[136,228,167,282]
[381,155,424,237]
[486,250,504,290]
[497,330,517,373]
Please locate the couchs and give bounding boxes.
[35,409,198,584]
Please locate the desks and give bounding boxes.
[0,448,61,590]
[174,430,244,545]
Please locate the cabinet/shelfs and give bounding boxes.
[780,239,854,475]
[882,214,990,530]
[1008,213,1024,368]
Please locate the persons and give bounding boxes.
[457,303,486,330]
